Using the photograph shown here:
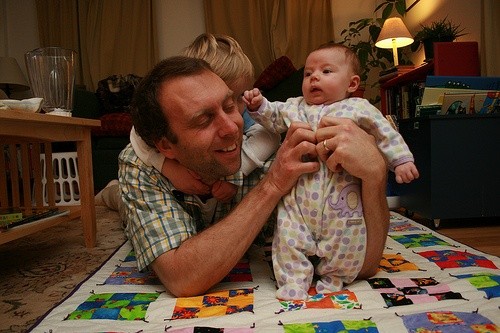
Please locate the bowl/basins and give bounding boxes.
[0,97,43,113]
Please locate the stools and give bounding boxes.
[38,152,81,205]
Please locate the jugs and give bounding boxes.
[25,46,78,113]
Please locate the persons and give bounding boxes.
[241,43,420,301]
[95,56,390,298]
[131,32,281,202]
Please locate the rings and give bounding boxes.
[323,139,329,150]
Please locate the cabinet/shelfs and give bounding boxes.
[379,41,500,225]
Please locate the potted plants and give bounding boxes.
[409,16,469,60]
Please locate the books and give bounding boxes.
[379,65,415,83]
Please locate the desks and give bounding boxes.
[0,107,101,256]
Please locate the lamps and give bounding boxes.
[376,18,415,67]
